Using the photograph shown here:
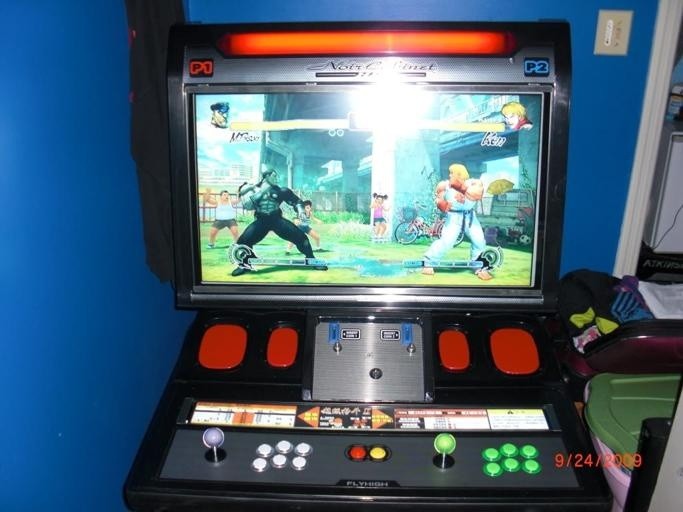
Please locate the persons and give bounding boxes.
[420,163,496,284]
[286,198,325,255]
[503,102,535,133]
[203,189,241,248]
[209,102,231,132]
[368,193,389,245]
[229,168,327,276]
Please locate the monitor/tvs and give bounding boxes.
[169,83,554,317]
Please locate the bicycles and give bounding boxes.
[394,200,465,249]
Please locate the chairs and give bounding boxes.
[586,317,683,512]
